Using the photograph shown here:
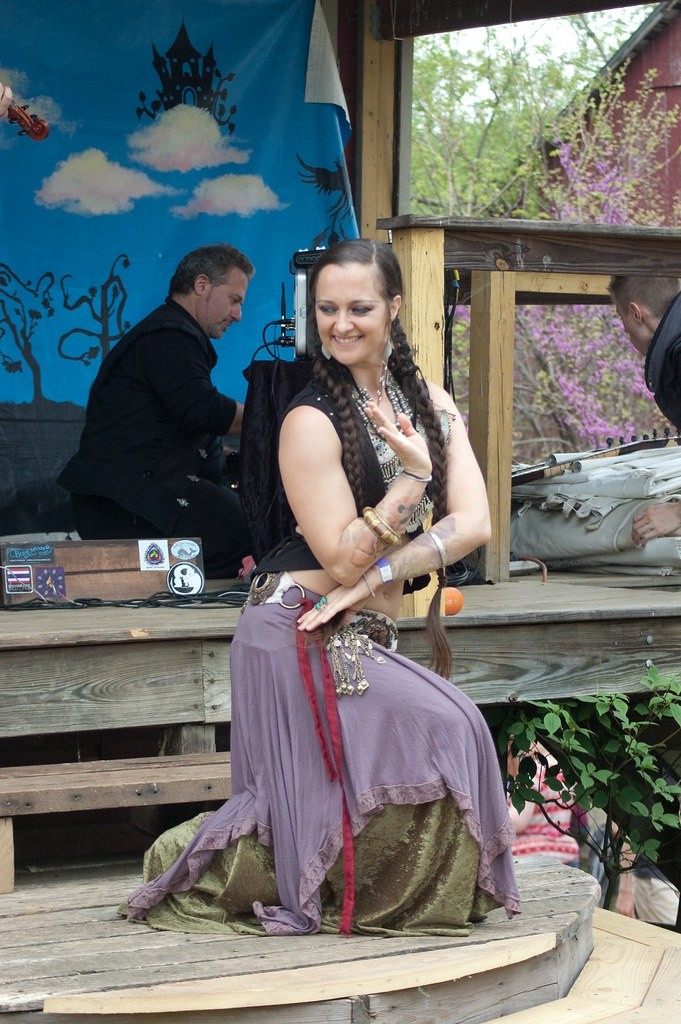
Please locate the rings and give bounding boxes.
[314,602,322,611]
[319,595,328,607]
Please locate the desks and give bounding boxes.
[1,580,681,757]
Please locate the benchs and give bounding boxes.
[0,751,240,895]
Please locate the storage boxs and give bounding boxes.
[1,537,206,608]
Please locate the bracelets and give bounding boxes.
[362,505,402,549]
[363,574,375,598]
[375,557,393,584]
[400,467,433,483]
[425,531,447,568]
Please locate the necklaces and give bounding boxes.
[343,370,416,440]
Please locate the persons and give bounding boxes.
[142,240,493,925]
[609,274,681,549]
[57,244,254,576]
[506,730,681,929]
